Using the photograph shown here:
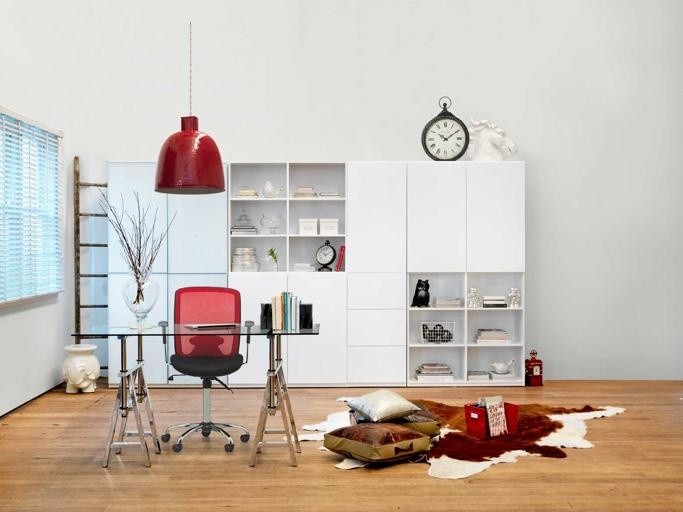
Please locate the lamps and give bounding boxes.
[153,20,225,195]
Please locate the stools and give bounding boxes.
[62,344,99,395]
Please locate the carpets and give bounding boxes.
[294,400,626,480]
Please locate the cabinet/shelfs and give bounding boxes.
[346,162,406,271]
[407,162,524,273]
[108,274,227,390]
[407,307,524,387]
[229,196,345,271]
[347,272,407,388]
[107,163,228,274]
[228,271,346,390]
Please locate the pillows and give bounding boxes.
[347,409,441,438]
[336,389,421,422]
[324,421,431,466]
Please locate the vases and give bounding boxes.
[121,271,156,323]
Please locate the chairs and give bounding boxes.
[162,286,250,452]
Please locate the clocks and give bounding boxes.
[420,96,469,161]
[314,240,336,272]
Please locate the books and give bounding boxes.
[229,186,340,235]
[413,294,516,381]
[269,289,301,329]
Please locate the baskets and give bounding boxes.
[416,322,457,345]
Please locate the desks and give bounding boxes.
[72,324,320,468]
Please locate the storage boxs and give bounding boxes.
[299,218,317,235]
[464,401,520,439]
[320,218,338,235]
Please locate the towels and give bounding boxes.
[486,395,508,438]
